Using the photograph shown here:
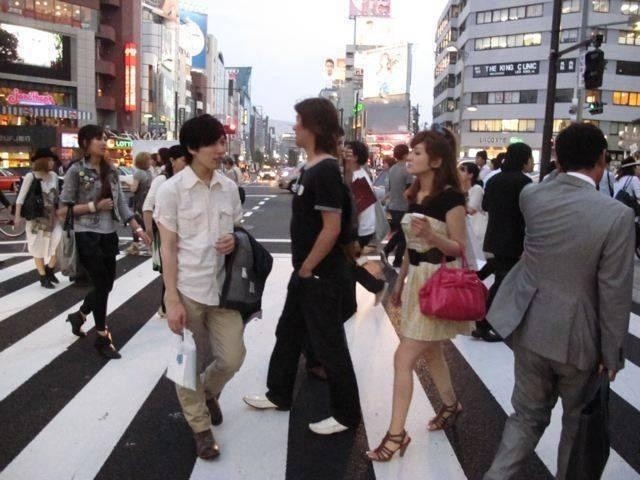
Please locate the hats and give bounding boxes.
[29,147,59,163]
[619,156,639,170]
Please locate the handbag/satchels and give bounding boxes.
[614,176,639,218]
[56,205,80,273]
[10,172,46,220]
[237,186,245,206]
[162,326,199,392]
[417,240,491,322]
[347,176,378,215]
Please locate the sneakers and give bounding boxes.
[380,248,390,266]
[470,328,504,344]
[125,243,154,258]
[205,396,224,426]
[37,265,79,291]
[191,429,220,460]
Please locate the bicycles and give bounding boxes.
[1,201,28,238]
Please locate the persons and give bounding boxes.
[1,112,247,459]
[320,58,336,89]
[243,99,640,479]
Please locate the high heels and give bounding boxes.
[64,311,88,342]
[93,331,121,362]
[364,427,411,468]
[424,399,464,434]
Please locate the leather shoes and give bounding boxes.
[308,416,349,435]
[240,393,279,414]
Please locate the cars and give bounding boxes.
[257,164,276,180]
[278,161,374,195]
[116,164,133,192]
[0,167,27,194]
[57,175,65,196]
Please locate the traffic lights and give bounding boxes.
[596,100,603,113]
[587,101,598,116]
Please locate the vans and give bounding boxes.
[455,159,490,171]
